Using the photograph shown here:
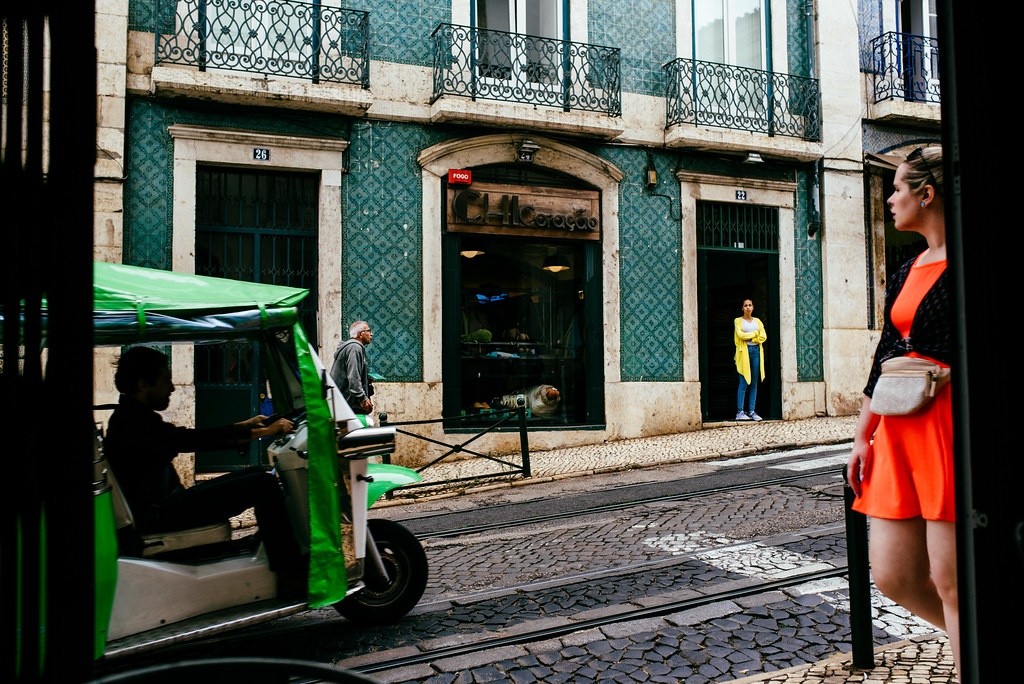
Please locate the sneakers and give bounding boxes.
[748,411,762,422]
[736,410,752,420]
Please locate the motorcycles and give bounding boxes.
[1,262,430,679]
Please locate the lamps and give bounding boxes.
[744,154,765,163]
[460,244,485,259]
[517,139,542,153]
[542,255,571,273]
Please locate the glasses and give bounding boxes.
[907,146,938,186]
[362,328,371,332]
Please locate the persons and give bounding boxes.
[733,299,768,422]
[103,345,309,601]
[330,321,373,429]
[846,146,962,684]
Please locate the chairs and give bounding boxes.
[96,441,232,560]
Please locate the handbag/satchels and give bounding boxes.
[869,356,951,415]
